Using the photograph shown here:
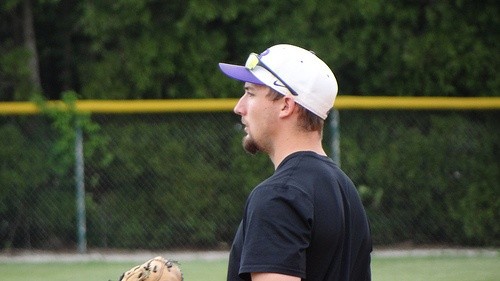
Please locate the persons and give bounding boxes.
[117,43,373,281]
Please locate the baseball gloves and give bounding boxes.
[119,256,183,281]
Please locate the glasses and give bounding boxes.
[244,52,299,97]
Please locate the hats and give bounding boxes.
[218,44,339,121]
